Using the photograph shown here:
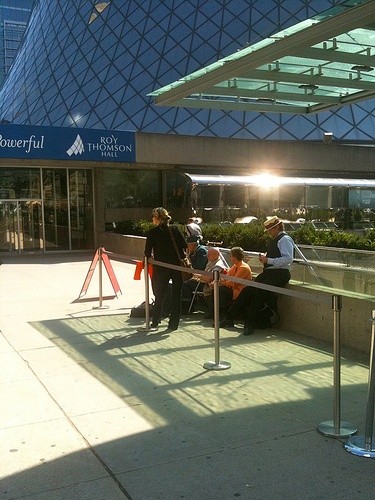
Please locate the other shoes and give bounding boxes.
[150,323,158,327]
[168,324,178,330]
[244,326,253,335]
[220,320,234,327]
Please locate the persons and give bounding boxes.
[213,215,294,336]
[211,247,252,320]
[144,207,188,329]
[193,247,226,319]
[187,236,208,311]
[187,219,201,244]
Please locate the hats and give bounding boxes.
[186,235,203,242]
[263,216,283,233]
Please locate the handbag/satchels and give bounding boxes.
[180,257,194,281]
